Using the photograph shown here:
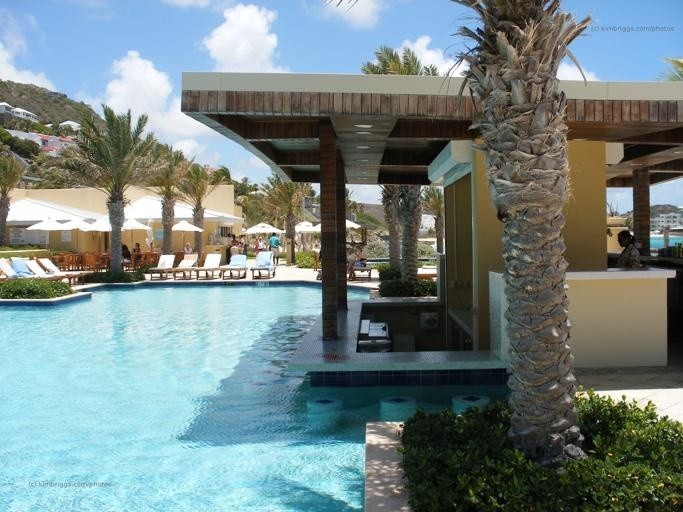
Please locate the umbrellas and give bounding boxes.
[124,218,150,258]
[245,222,284,249]
[28,220,71,251]
[64,215,88,249]
[88,215,125,255]
[146,215,154,252]
[284,221,315,241]
[172,220,205,251]
[311,218,361,233]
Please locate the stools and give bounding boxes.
[351,264,372,280]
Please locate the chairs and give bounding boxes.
[0,236,278,285]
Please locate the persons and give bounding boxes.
[133,243,143,263]
[665,225,670,248]
[121,244,130,264]
[184,242,192,254]
[617,230,644,267]
[227,233,280,265]
[346,247,366,281]
